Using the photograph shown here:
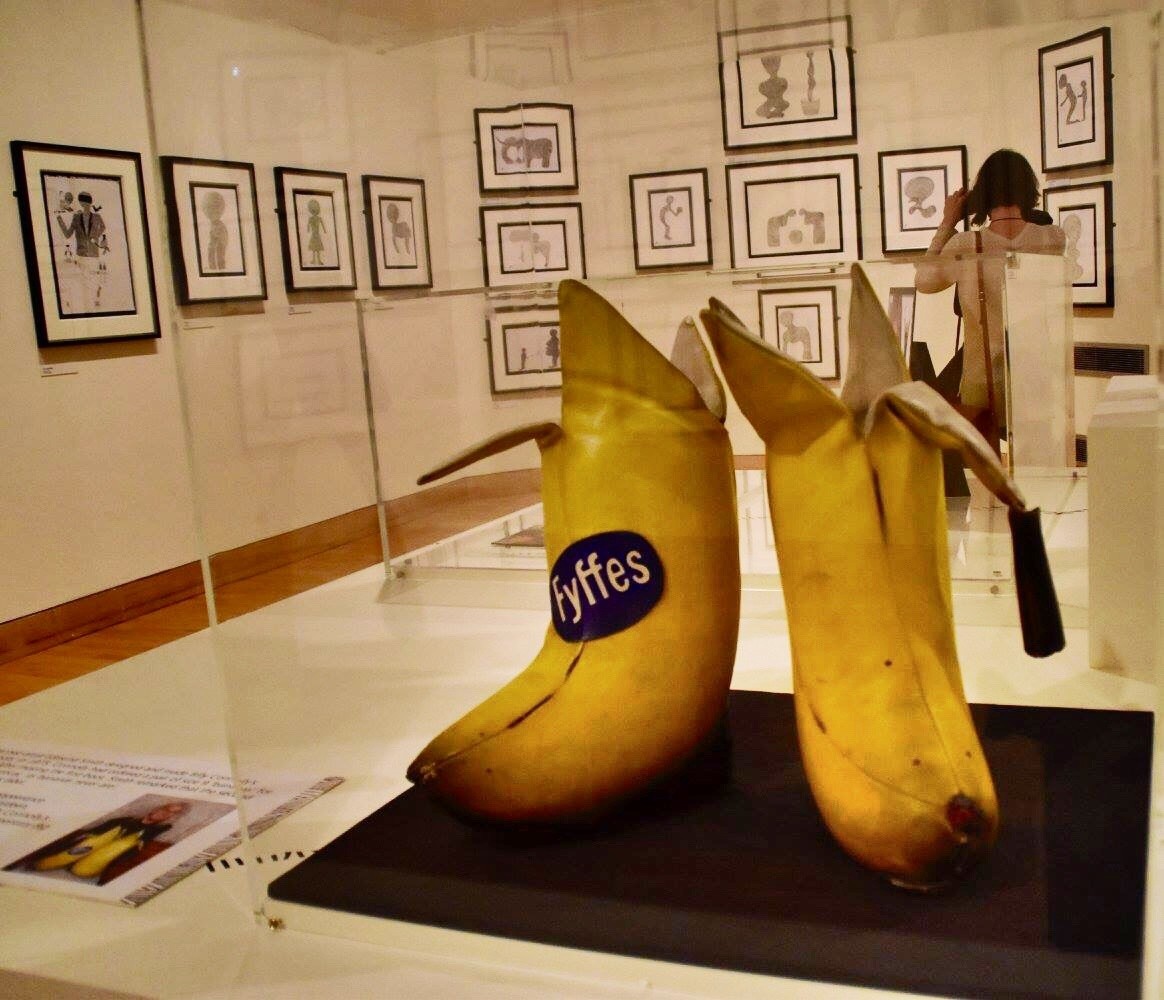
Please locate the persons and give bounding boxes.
[916,150,1065,438]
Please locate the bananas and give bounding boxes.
[36,825,144,876]
[697,263,1064,882]
[406,275,740,820]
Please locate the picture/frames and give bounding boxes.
[716,18,858,154]
[160,157,268,306]
[757,286,841,381]
[274,165,358,293]
[485,305,562,395]
[474,103,578,192]
[627,168,713,270]
[479,203,586,287]
[471,30,574,86]
[878,145,969,256]
[1042,180,1113,309]
[725,154,861,270]
[363,174,431,290]
[1040,26,1113,174]
[10,139,162,346]
